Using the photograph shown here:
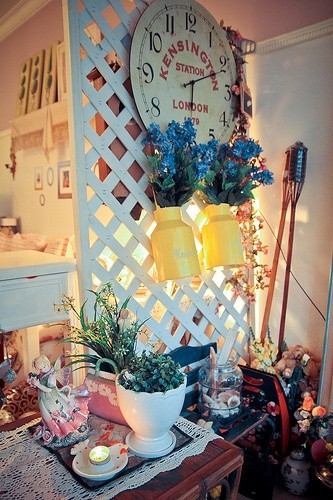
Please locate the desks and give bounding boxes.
[0,250,78,387]
[0,396,244,500]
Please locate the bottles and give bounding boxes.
[197,355,243,419]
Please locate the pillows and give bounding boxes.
[0,232,75,258]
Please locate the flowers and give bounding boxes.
[220,18,273,301]
[121,350,188,394]
[47,277,153,373]
[294,396,333,465]
[245,325,321,399]
[196,139,274,206]
[143,121,217,208]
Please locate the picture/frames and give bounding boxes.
[57,160,72,198]
[55,21,103,101]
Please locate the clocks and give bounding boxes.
[128,0,238,148]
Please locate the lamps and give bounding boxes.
[261,142,309,360]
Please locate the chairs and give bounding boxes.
[162,342,276,443]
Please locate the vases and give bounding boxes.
[202,203,245,270]
[116,372,187,441]
[153,207,201,281]
